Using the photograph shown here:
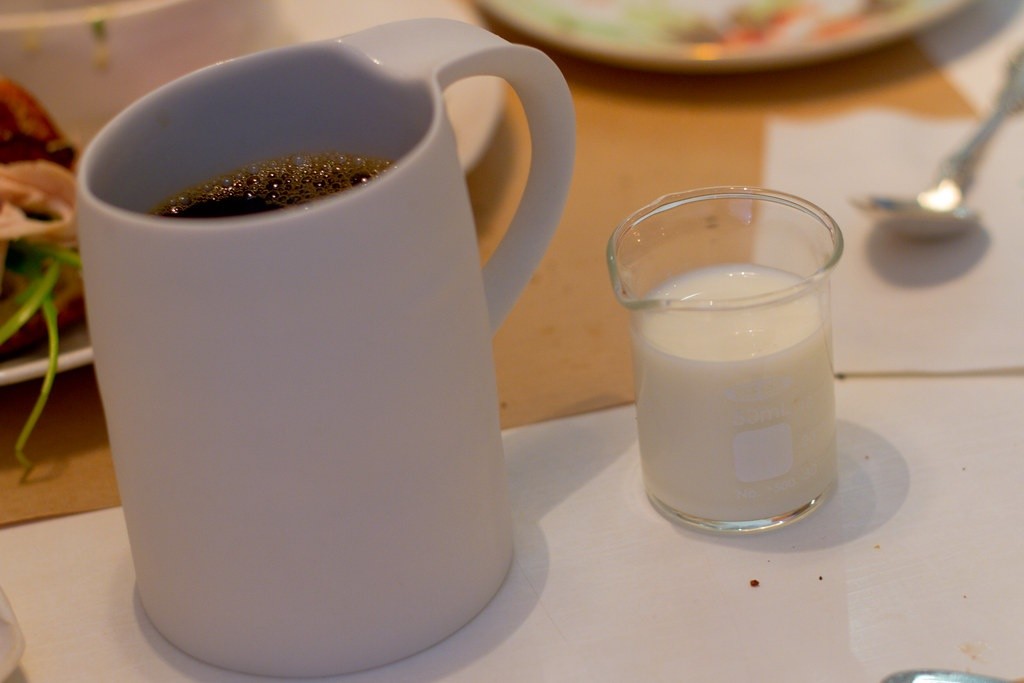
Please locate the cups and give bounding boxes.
[607,184,845,536]
[72,19,575,683]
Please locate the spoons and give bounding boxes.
[852,41,1024,243]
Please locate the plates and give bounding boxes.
[467,0,973,73]
[0,335,93,397]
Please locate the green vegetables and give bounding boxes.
[0,238,82,483]
